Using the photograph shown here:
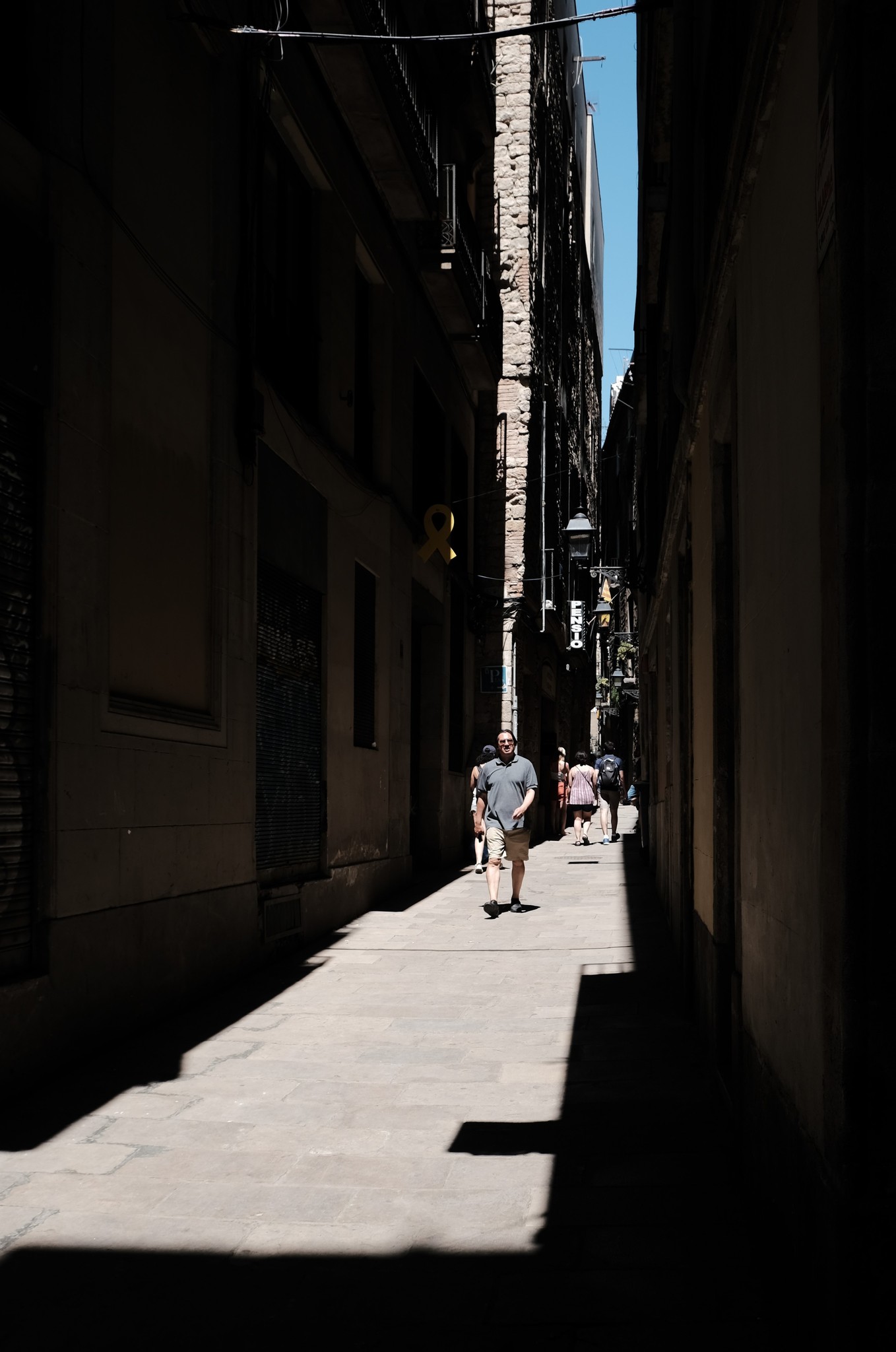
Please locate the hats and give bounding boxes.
[483,745,496,754]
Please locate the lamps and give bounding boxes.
[611,666,639,698]
[592,597,639,646]
[560,500,630,587]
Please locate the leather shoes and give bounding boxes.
[484,901,499,917]
[510,898,522,912]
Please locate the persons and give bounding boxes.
[474,729,538,917]
[569,750,597,846]
[547,747,570,835]
[471,745,503,874]
[594,741,640,846]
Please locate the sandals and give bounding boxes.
[575,841,581,846]
[583,834,590,845]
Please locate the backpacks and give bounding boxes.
[599,757,620,791]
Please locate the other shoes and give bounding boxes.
[560,829,570,836]
[603,838,610,845]
[475,863,484,873]
[500,862,503,869]
[612,834,620,842]
